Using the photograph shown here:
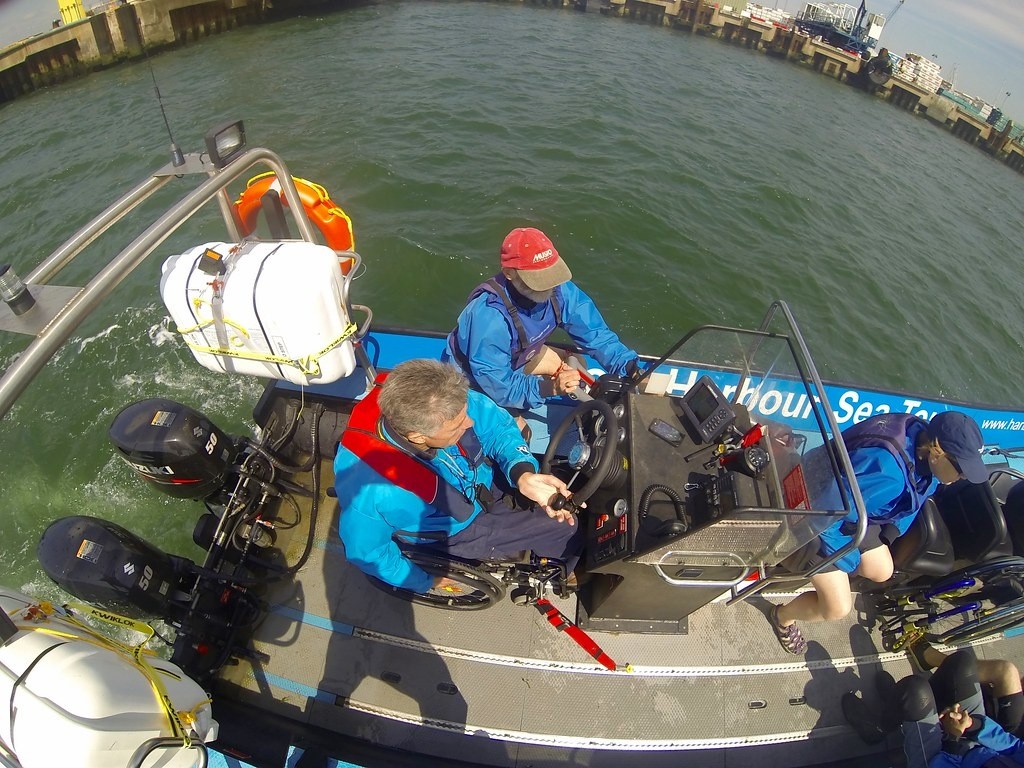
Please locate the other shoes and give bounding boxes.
[556,568,593,587]
[875,670,896,699]
[841,692,888,745]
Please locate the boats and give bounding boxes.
[0,25,1024,768]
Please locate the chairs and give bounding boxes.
[776,448,1024,594]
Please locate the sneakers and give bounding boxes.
[908,636,934,674]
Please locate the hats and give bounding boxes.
[501,228,572,291]
[929,411,989,484]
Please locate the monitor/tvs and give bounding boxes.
[687,385,718,422]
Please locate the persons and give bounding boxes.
[771,410,989,656]
[842,618,1024,768]
[333,360,591,593]
[447,227,666,450]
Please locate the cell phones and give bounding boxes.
[648,418,685,448]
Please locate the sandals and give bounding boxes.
[771,603,808,656]
